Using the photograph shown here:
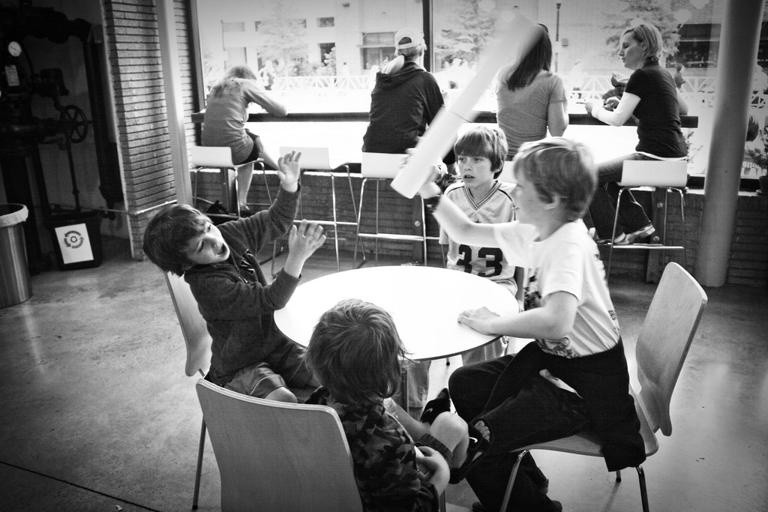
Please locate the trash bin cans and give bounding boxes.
[0,203,33,309]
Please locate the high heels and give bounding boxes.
[238,206,250,218]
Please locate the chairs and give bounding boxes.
[606,160,688,287]
[188,145,274,219]
[194,379,371,511]
[353,152,448,267]
[162,260,316,510]
[498,164,520,223]
[499,261,708,510]
[271,143,366,278]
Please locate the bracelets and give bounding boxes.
[423,192,442,214]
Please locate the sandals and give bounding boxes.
[450,418,493,484]
[421,389,450,425]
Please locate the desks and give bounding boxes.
[191,96,701,284]
[270,262,524,424]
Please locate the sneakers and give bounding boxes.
[589,227,626,246]
[620,222,655,244]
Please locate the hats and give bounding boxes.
[394,28,424,49]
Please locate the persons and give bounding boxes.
[583,22,689,246]
[491,20,569,160]
[360,25,458,191]
[435,123,528,369]
[300,298,496,512]
[673,63,688,93]
[141,145,450,445]
[197,63,287,213]
[401,136,631,511]
